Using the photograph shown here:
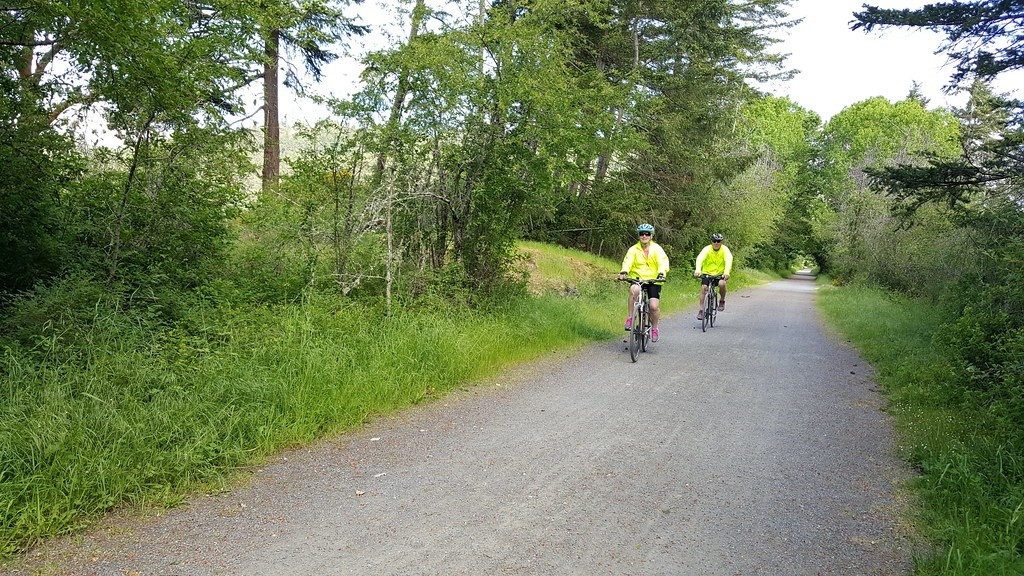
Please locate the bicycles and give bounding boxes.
[614,276,668,363]
[692,273,728,332]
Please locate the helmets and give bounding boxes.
[636,224,654,234]
[711,233,723,240]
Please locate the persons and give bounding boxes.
[616,224,670,343]
[694,233,733,321]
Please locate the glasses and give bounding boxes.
[639,232,651,236]
[713,240,721,243]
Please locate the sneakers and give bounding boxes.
[696,309,704,320]
[625,316,632,330]
[718,300,725,311]
[652,327,659,342]
